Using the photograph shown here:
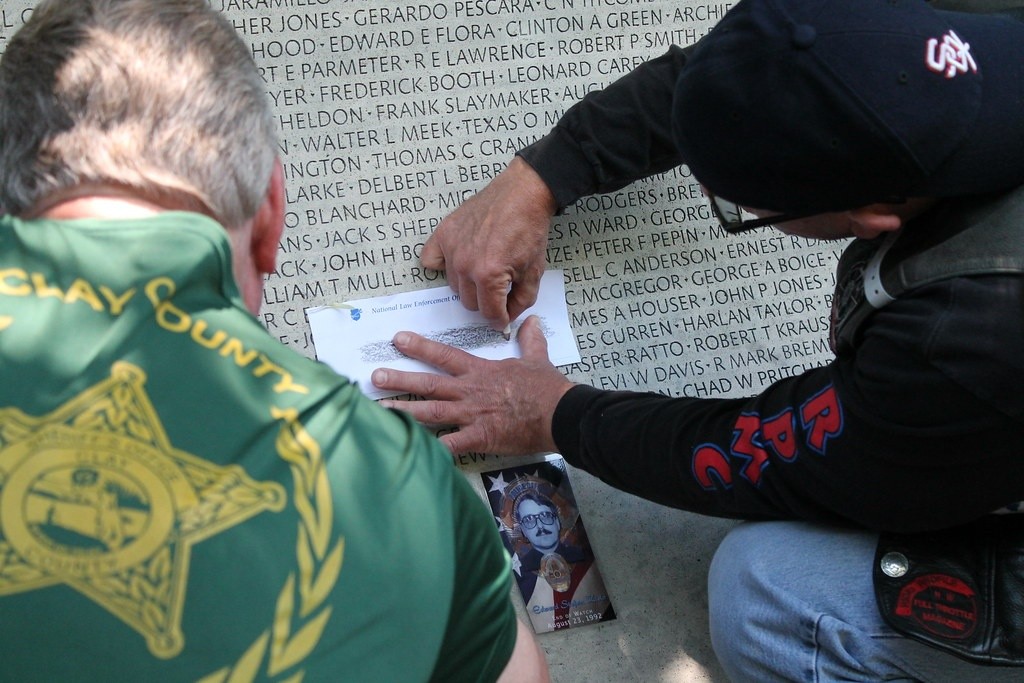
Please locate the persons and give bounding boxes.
[0,0,550,683]
[371,0,1024,683]
[515,491,586,572]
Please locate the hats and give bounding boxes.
[670,0,1024,217]
[499,475,579,538]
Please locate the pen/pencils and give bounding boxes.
[503,323,512,341]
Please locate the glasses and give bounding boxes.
[521,511,559,529]
[709,194,834,234]
